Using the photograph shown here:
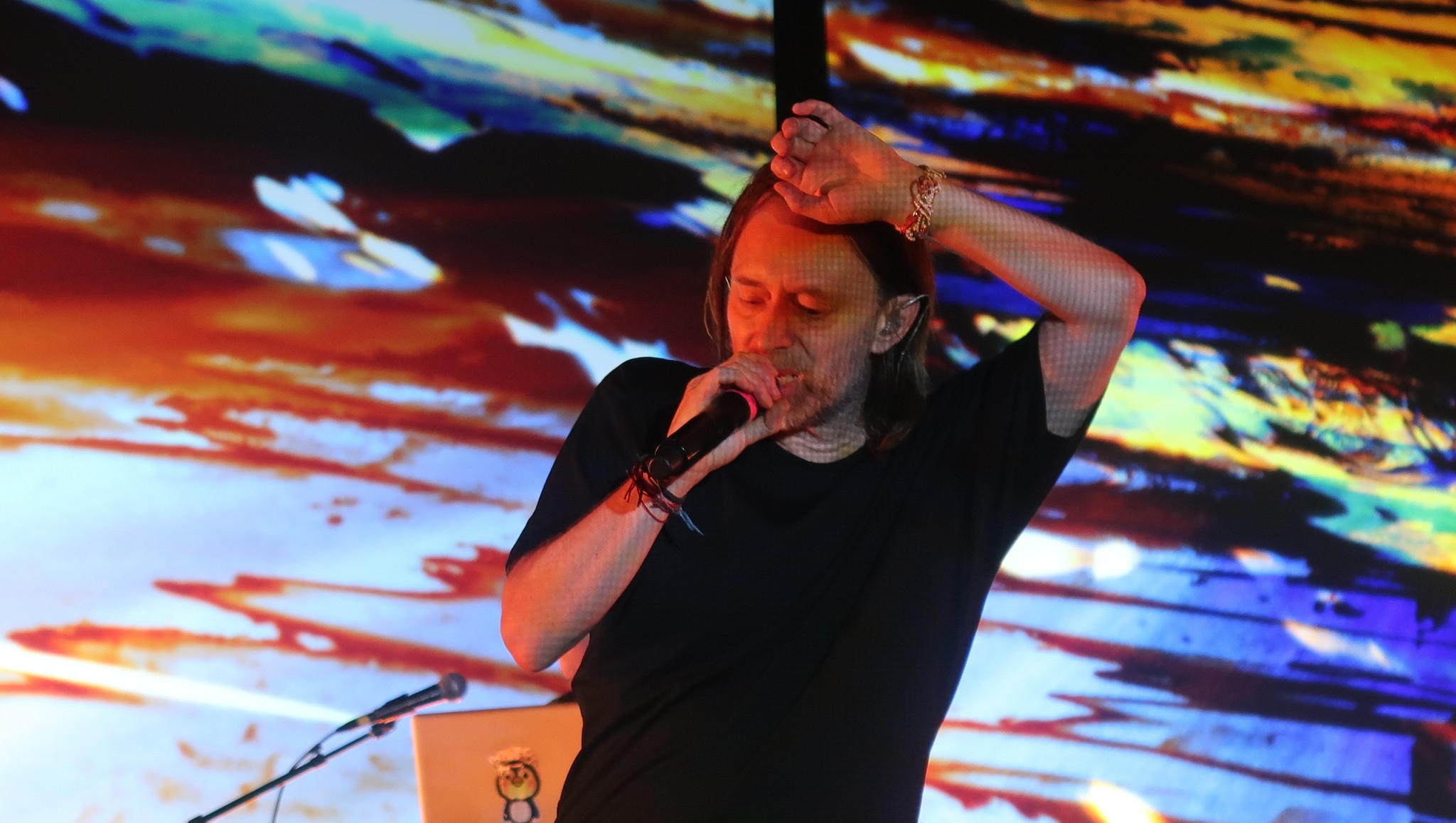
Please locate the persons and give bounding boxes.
[503,100,1148,823]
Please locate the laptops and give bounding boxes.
[411,702,583,823]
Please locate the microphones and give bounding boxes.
[645,384,766,480]
[336,672,468,733]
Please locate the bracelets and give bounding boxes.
[629,455,705,537]
[895,164,947,242]
